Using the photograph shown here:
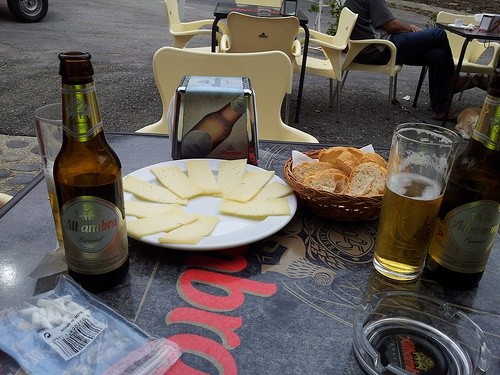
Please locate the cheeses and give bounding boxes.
[124,158,293,243]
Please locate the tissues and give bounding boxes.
[474,13,500,33]
[165,74,260,167]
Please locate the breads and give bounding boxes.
[296,147,388,194]
[456,107,483,139]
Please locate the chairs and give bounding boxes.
[134,0,500,143]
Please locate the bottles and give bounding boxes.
[52,50,128,289]
[181,93,250,161]
[424,70,500,288]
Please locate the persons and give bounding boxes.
[344,0,477,120]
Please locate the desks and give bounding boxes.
[411,22,500,128]
[0,130,500,375]
[212,1,311,124]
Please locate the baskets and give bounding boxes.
[284,148,384,222]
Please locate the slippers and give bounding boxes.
[452,72,484,96]
[431,111,454,122]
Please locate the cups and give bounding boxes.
[454,19,463,26]
[32,102,63,244]
[371,121,460,281]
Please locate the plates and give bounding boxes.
[449,24,467,28]
[122,158,297,251]
